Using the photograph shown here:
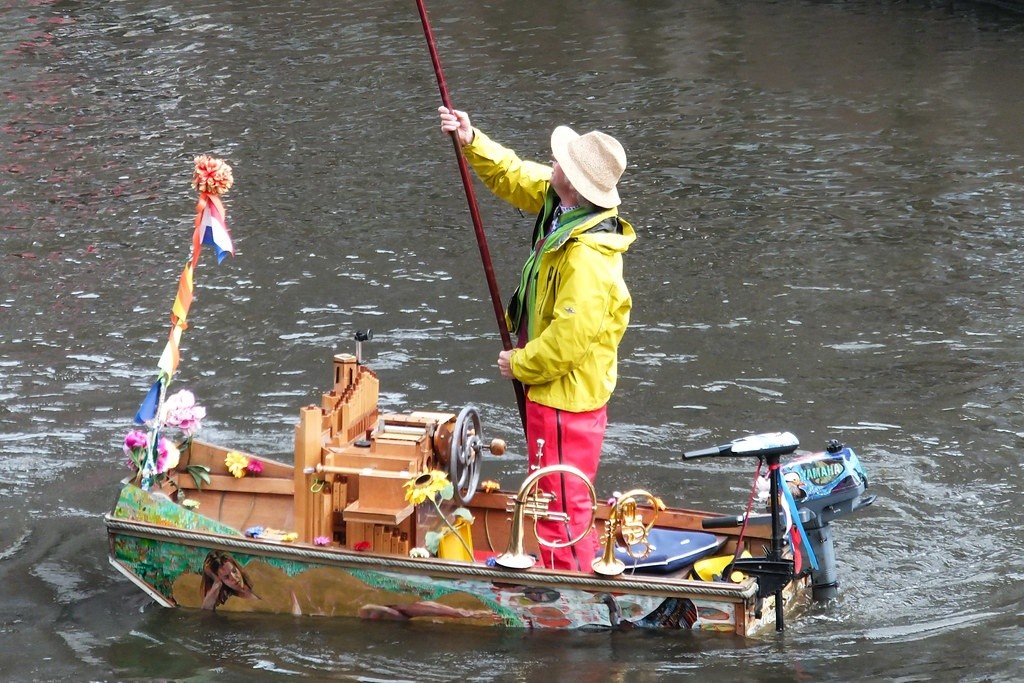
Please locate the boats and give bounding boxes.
[101,327,876,638]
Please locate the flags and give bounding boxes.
[196,197,238,264]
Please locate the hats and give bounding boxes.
[551,125,627,208]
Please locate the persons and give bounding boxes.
[439,105,636,574]
[199,551,504,623]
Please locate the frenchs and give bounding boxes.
[494,438,598,569]
[591,488,659,575]
